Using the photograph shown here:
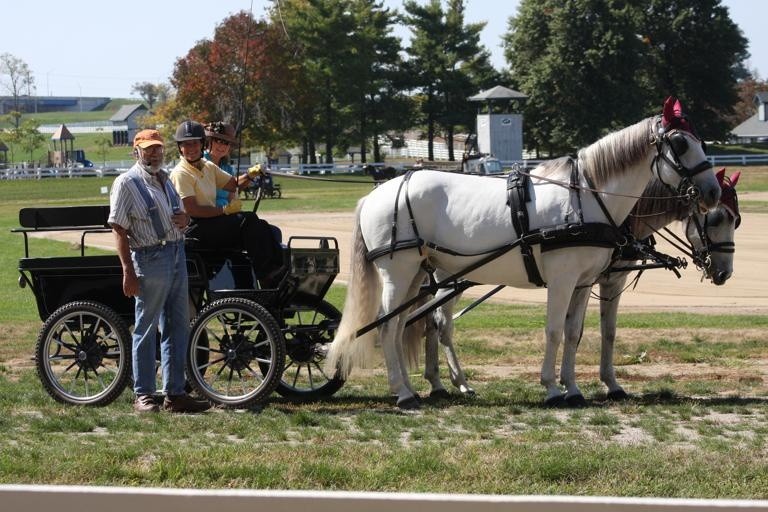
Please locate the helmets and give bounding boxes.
[174,121,206,142]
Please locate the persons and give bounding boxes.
[204,121,235,208]
[413,158,423,167]
[169,120,283,289]
[107,128,211,414]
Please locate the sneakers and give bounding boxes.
[262,263,289,289]
[135,395,161,413]
[163,395,211,412]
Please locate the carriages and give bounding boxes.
[8,93,754,410]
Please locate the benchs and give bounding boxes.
[178,235,249,266]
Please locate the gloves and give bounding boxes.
[223,198,243,216]
[246,164,264,180]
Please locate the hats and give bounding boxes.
[204,121,238,145]
[133,129,165,149]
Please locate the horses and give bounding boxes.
[401,168,740,401]
[323,95,722,409]
[363,165,397,189]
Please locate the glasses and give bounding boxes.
[212,136,230,145]
